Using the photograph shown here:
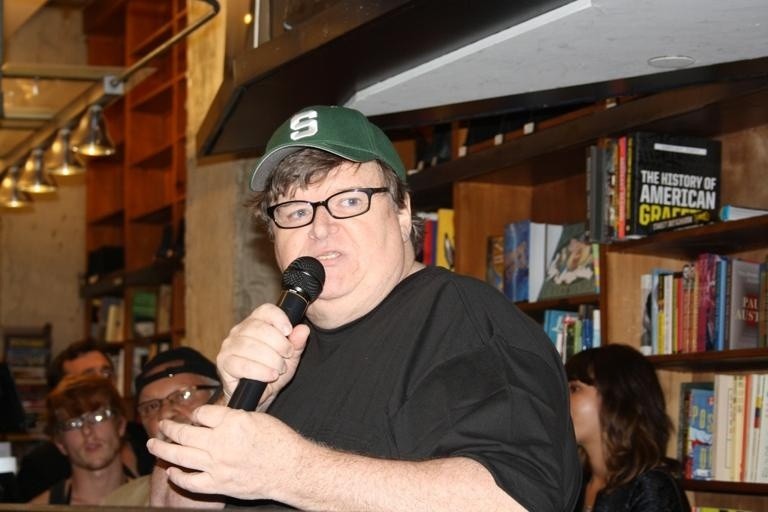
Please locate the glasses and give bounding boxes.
[267,186,393,229]
[136,384,221,414]
[55,407,116,430]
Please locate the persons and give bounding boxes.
[103,347,224,507]
[20,336,156,502]
[565,341,692,511]
[142,105,583,509]
[21,371,136,505]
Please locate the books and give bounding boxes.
[413,206,456,269]
[669,371,767,487]
[132,343,169,392]
[7,336,45,365]
[479,214,598,303]
[584,132,722,243]
[12,367,49,385]
[21,398,49,432]
[635,249,767,352]
[108,349,125,397]
[132,284,167,335]
[540,304,600,365]
[90,296,123,341]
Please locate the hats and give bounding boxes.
[136,347,221,391]
[249,106,407,193]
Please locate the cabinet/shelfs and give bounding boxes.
[85,2,188,423]
[405,108,605,365]
[606,56,766,511]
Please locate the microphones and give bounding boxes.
[226,256,327,414]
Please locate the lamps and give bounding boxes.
[1,99,118,211]
